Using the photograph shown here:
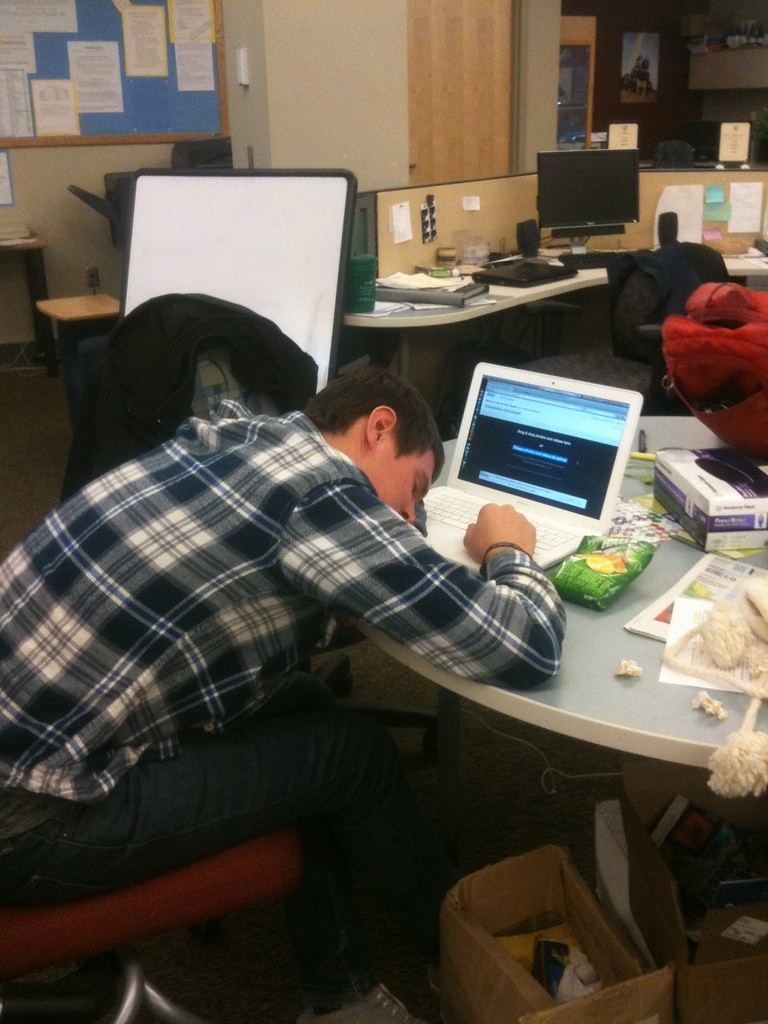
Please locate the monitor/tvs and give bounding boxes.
[537,148,640,253]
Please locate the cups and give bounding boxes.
[343,251,377,313]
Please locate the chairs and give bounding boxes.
[89,294,437,818]
[509,242,731,414]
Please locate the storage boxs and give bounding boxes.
[440,762,768,1024]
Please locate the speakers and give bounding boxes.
[658,212,679,246]
[517,220,541,259]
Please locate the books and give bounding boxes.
[625,552,768,696]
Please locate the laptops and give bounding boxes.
[471,259,578,289]
[425,362,644,573]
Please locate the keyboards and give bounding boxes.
[559,252,616,270]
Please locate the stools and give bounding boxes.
[0,829,303,1024]
[37,293,120,436]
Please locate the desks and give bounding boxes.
[344,248,768,383]
[344,417,768,770]
[0,234,60,377]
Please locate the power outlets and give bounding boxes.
[84,265,100,288]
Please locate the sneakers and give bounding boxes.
[298,982,421,1024]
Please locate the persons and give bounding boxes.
[0,369,568,1024]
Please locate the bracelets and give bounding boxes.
[477,543,535,580]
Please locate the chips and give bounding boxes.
[574,551,627,574]
